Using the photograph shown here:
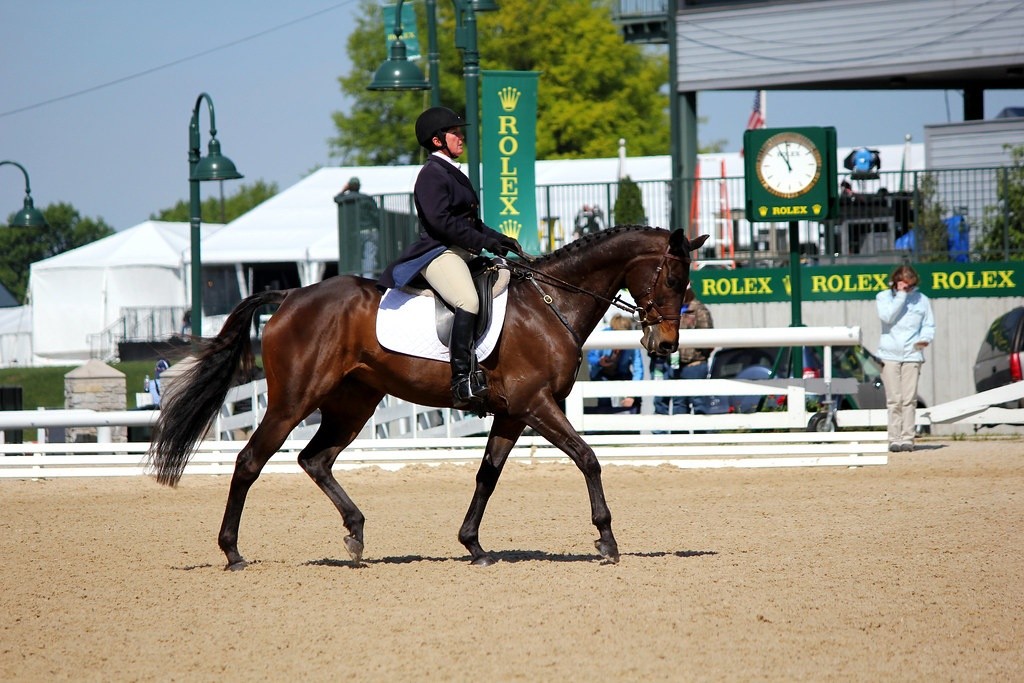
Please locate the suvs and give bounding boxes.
[973,304,1023,409]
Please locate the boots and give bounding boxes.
[451,307,488,401]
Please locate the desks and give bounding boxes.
[712,210,779,259]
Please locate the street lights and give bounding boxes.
[187,94,245,360]
[368,1,500,220]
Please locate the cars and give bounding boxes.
[653,343,930,437]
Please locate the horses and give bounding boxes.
[152,221,709,568]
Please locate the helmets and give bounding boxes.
[416,106,471,145]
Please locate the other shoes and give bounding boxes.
[901,444,913,452]
[890,444,900,452]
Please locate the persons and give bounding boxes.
[146,358,170,408]
[586,285,714,435]
[875,265,933,453]
[229,352,266,441]
[383,108,528,406]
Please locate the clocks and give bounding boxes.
[755,131,823,199]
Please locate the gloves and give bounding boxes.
[483,237,508,256]
[498,236,521,253]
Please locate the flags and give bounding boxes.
[741,90,762,157]
